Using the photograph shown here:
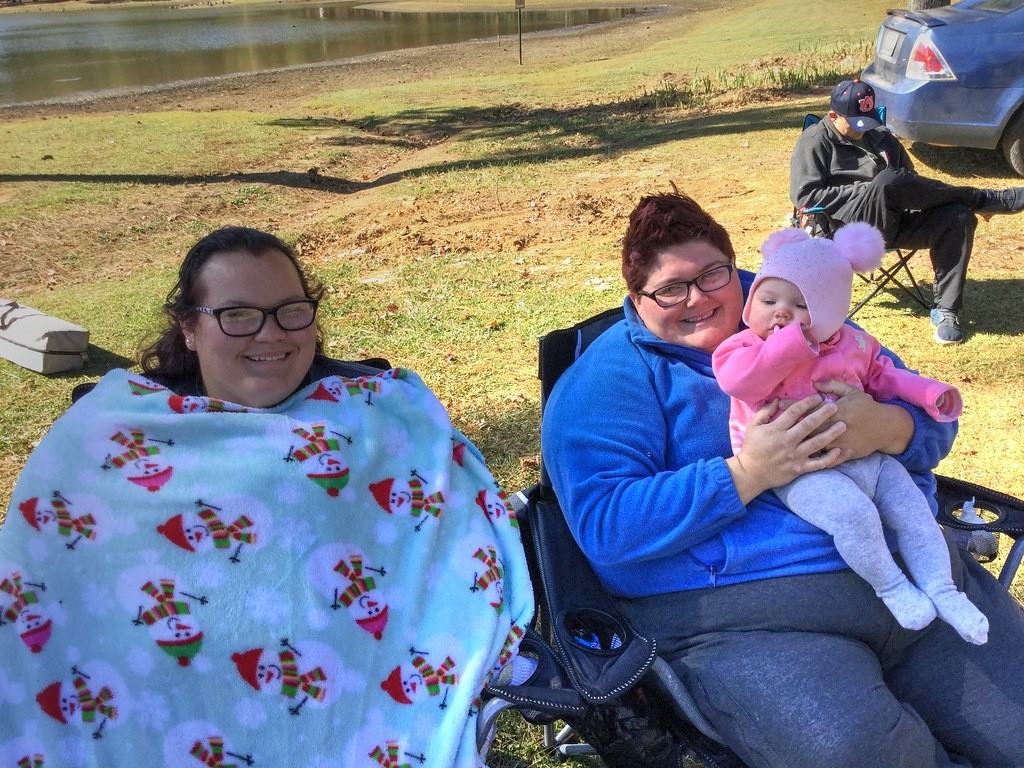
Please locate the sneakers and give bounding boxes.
[930,307,965,346]
[974,187,1023,215]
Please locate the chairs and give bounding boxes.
[790,105,931,320]
[508,307,1024,768]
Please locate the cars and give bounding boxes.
[860,0,1024,177]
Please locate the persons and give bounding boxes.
[790,79,1024,343]
[542,180,1024,768]
[73,227,393,407]
[712,222,989,644]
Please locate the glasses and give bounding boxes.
[635,257,735,308]
[195,293,319,338]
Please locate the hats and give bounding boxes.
[885,283,888,285]
[830,79,883,132]
[742,221,886,343]
